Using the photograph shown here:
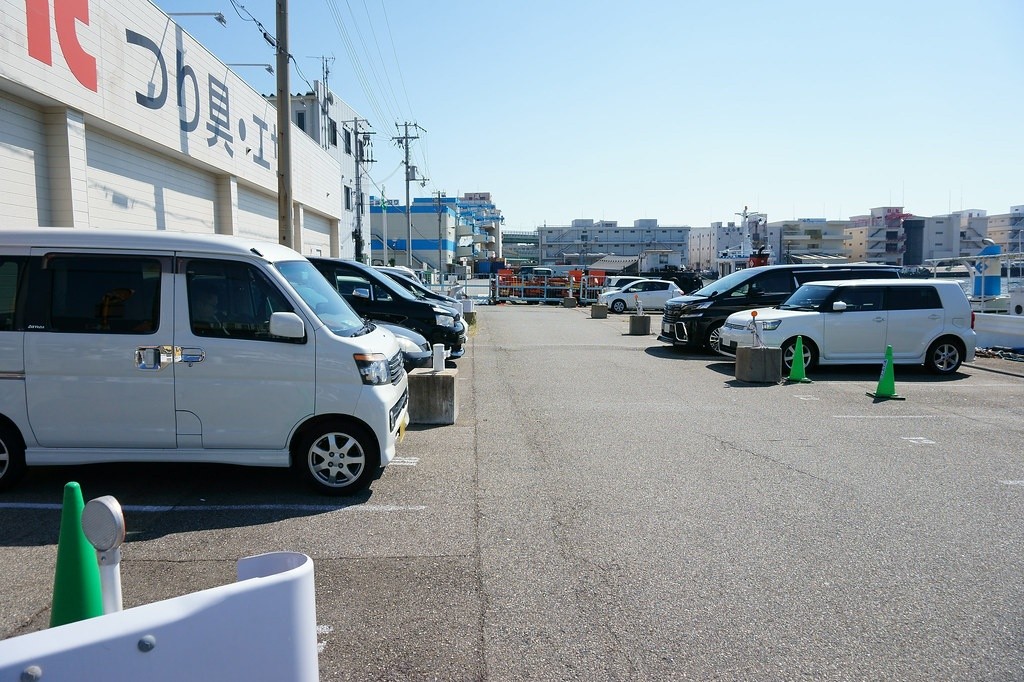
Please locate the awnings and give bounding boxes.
[586,255,639,272]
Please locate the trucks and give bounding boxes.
[528,267,556,283]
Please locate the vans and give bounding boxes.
[656,262,902,353]
[378,270,464,317]
[601,276,660,295]
[0,226,410,499]
[306,257,466,360]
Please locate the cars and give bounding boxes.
[600,279,685,313]
[337,276,391,300]
[360,316,433,370]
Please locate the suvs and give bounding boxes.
[718,279,975,374]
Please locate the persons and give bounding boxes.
[669,274,703,293]
[387,258,395,267]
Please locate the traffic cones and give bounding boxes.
[49,480,103,628]
[782,335,812,382]
[866,344,906,400]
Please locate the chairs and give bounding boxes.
[189,279,222,335]
